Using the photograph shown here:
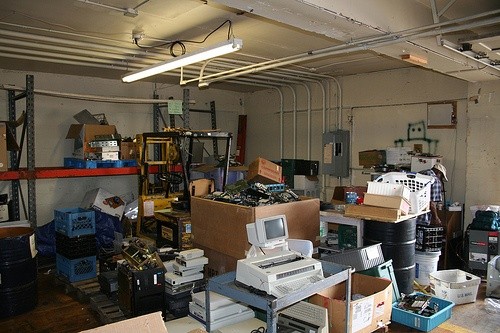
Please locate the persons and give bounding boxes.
[415,164,448,251]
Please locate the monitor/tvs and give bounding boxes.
[246,215,289,248]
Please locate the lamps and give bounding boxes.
[121,38,244,84]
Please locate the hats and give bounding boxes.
[434,163,448,182]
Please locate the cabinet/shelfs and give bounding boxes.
[0,157,241,272]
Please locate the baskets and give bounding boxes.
[376,171,434,214]
[251,183,284,192]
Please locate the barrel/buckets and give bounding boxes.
[415,251,442,285]
[363,218,417,294]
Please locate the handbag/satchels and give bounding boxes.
[469,210,498,231]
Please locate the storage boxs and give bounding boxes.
[308,273,393,333]
[65,109,137,169]
[117,247,255,331]
[55,209,98,282]
[80,189,126,220]
[359,259,400,303]
[247,159,282,184]
[192,195,319,258]
[321,242,384,270]
[205,167,242,191]
[0,122,9,173]
[363,172,436,211]
[429,268,482,304]
[393,292,455,330]
